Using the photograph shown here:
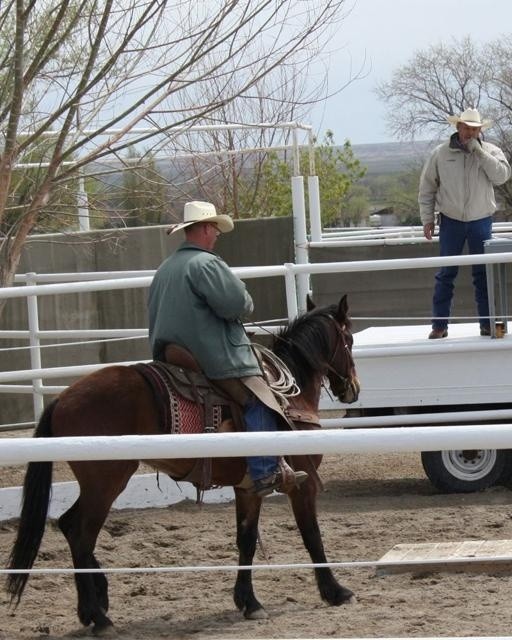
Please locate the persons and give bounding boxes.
[147,199,309,498]
[417,108,512,339]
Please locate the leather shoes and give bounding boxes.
[480,322,491,336]
[429,327,448,339]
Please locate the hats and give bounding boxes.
[166,201,235,236]
[448,107,493,131]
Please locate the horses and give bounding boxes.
[5,291,363,638]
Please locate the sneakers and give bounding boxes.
[256,467,309,497]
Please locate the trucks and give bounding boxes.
[317,321,512,493]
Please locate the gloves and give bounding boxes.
[463,136,481,153]
